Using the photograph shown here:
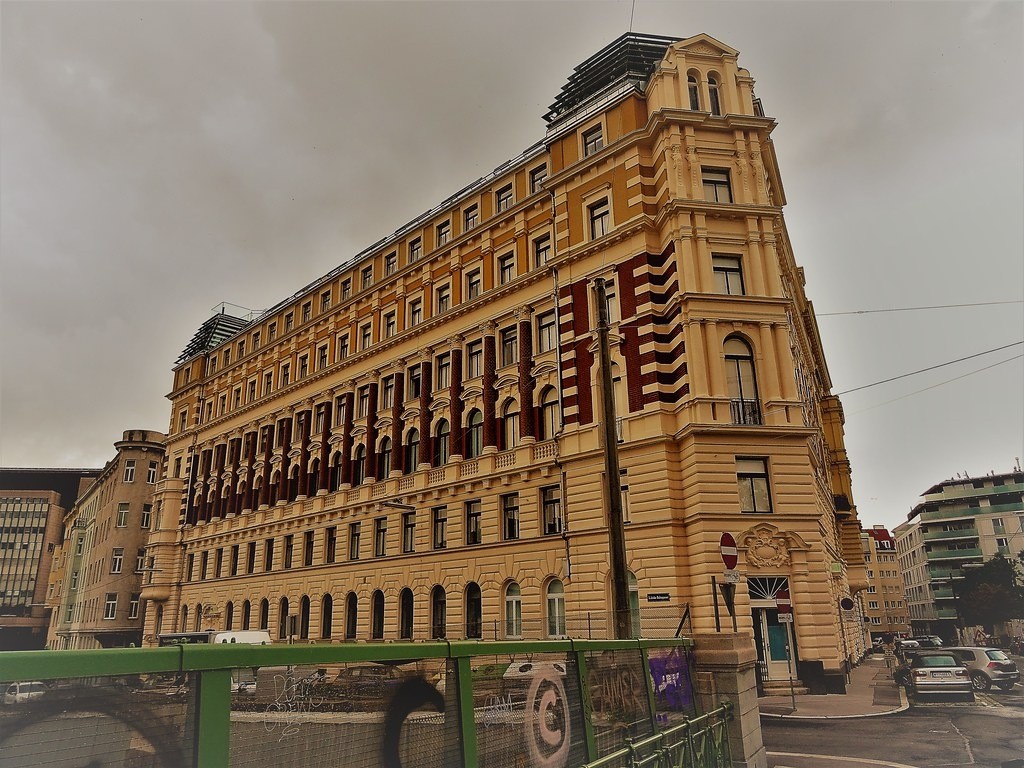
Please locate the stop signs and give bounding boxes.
[721,533,738,570]
[777,590,791,612]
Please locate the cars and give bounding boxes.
[909,650,973,701]
[4,681,50,705]
[872,637,882,646]
[308,666,405,713]
[896,641,919,650]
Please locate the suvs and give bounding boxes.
[913,635,943,647]
[935,647,1021,692]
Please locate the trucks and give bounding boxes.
[158,630,273,710]
